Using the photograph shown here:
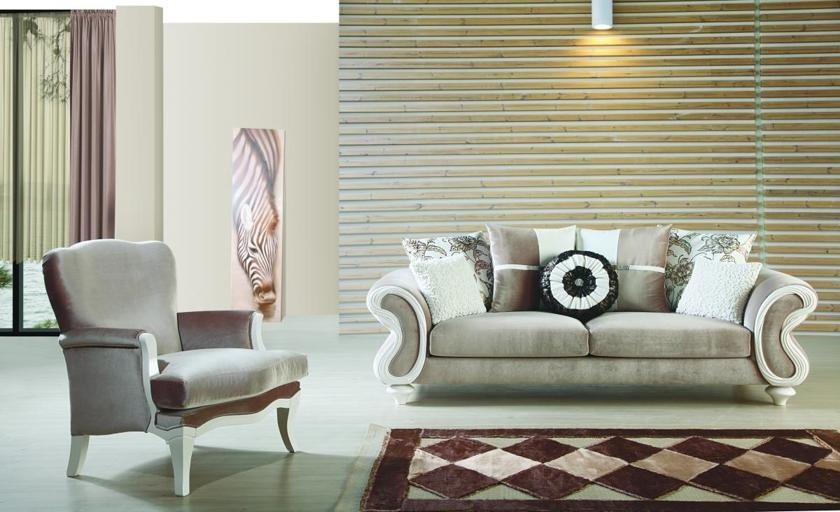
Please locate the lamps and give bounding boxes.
[592,0,614,29]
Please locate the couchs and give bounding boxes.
[363,261,820,406]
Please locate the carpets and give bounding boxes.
[358,427,840,512]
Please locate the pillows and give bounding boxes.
[401,230,496,324]
[664,227,759,310]
[578,224,670,313]
[540,249,620,322]
[483,224,576,315]
[675,259,762,326]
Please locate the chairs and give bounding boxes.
[42,238,311,496]
